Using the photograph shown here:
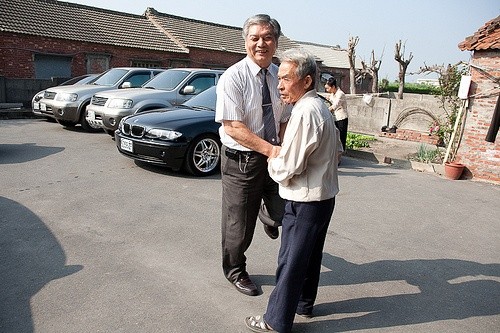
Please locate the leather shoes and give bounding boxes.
[232,276,259,296]
[263,222,279,239]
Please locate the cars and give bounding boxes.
[113,84,335,178]
[30,73,102,123]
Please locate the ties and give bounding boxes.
[260,68,277,145]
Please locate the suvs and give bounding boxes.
[39,67,168,133]
[85,66,229,137]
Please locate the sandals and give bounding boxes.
[244,314,277,333]
[297,311,312,319]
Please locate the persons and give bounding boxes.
[324,76,349,166]
[215,12,296,295]
[243,45,343,333]
